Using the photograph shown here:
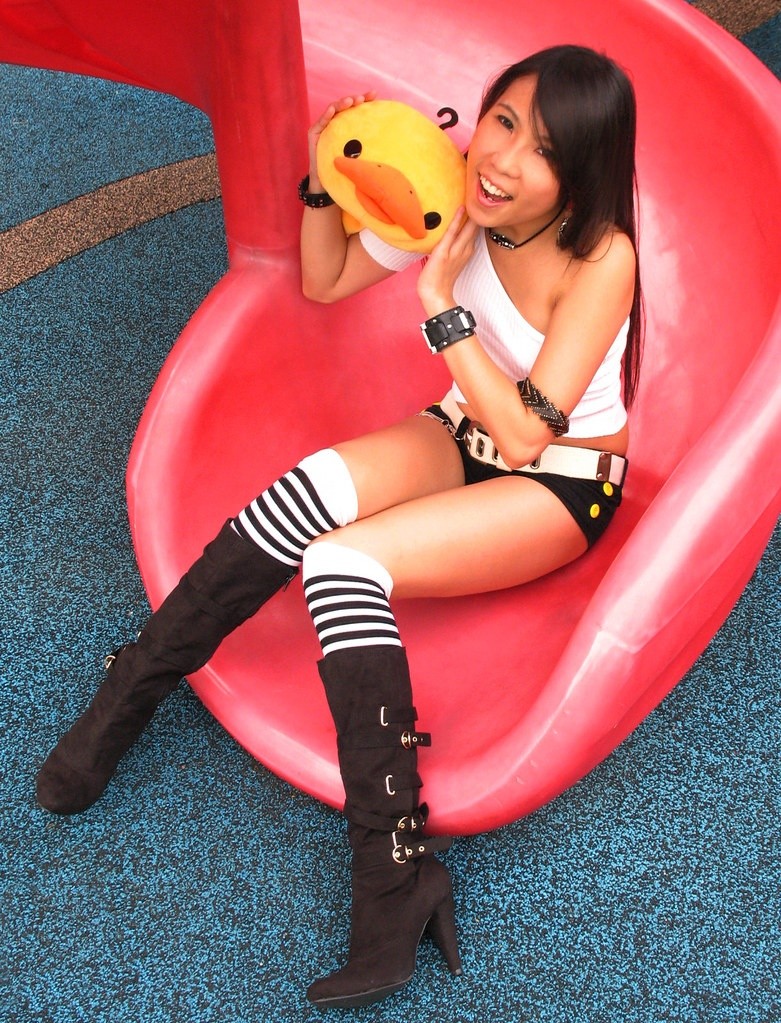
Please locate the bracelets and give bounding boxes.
[517,377,569,438]
[298,174,335,208]
[420,306,476,355]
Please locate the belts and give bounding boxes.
[440,394,625,487]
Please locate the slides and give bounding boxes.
[0,0,781,837]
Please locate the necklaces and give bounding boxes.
[488,200,566,251]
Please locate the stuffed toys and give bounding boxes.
[315,100,466,254]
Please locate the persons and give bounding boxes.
[36,43,648,1009]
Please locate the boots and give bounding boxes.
[306,645,463,1005]
[36,517,299,813]
